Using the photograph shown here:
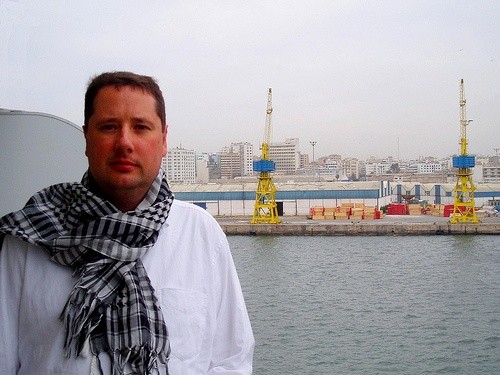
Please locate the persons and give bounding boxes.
[0,71,255,375]
